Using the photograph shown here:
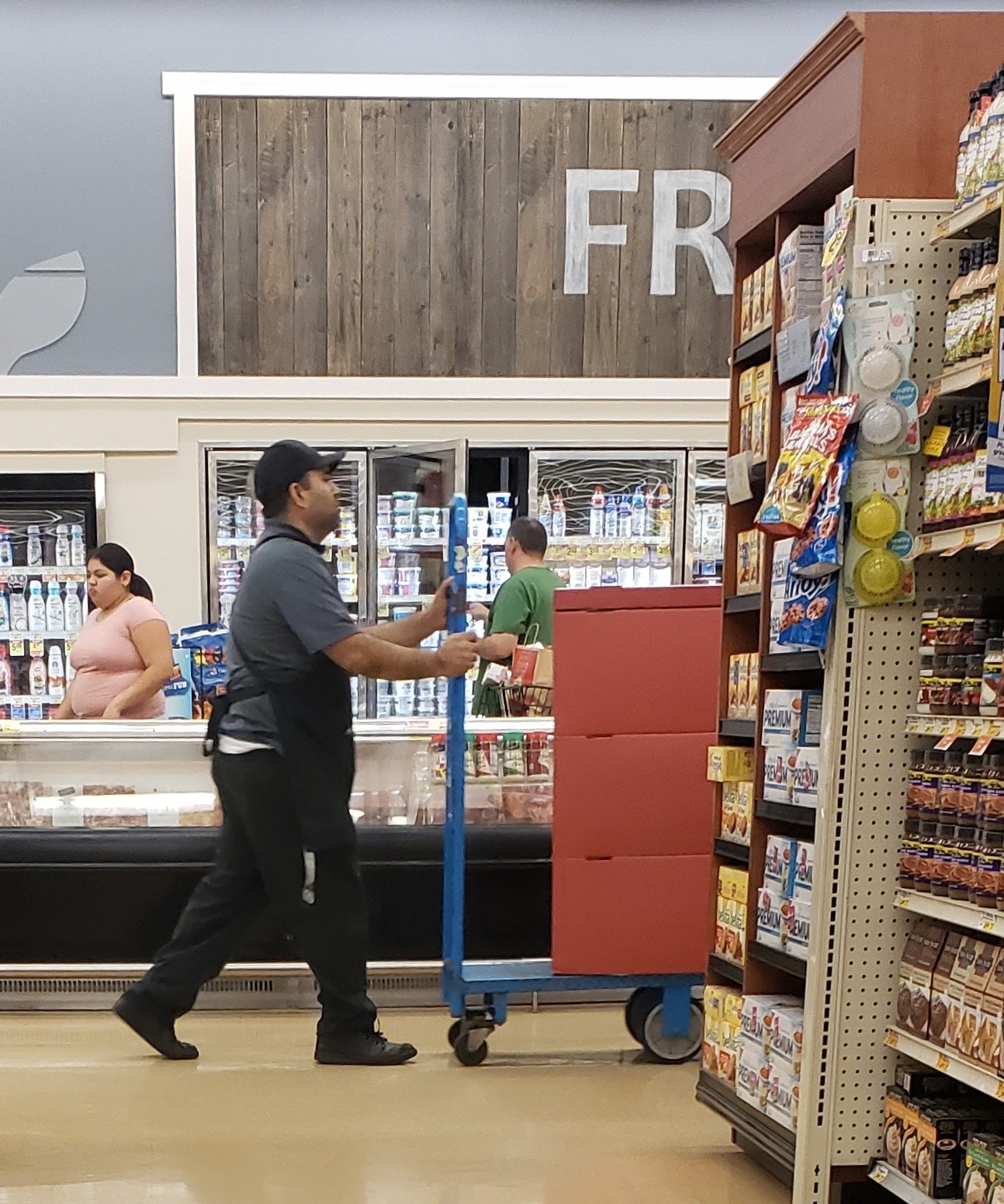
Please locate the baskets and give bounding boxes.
[484,682,553,717]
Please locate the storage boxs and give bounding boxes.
[550,527,836,978]
[737,224,824,346]
[703,984,740,1021]
[739,993,802,1046]
[765,1005,805,1080]
[764,1066,799,1133]
[468,507,489,525]
[722,991,745,1027]
[736,360,770,463]
[733,1043,773,1116]
[377,489,448,720]
[701,1014,722,1045]
[467,549,511,601]
[700,1038,721,1074]
[467,524,489,538]
[719,1021,740,1052]
[489,508,513,525]
[486,491,512,508]
[884,1062,1004,1204]
[716,1048,740,1085]
[490,524,511,538]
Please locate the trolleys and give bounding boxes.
[439,496,708,1066]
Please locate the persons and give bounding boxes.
[50,542,174,720]
[111,440,480,1065]
[469,516,567,719]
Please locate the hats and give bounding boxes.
[253,440,347,506]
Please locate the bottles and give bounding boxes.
[954,64,1004,213]
[921,402,1003,534]
[539,494,566,537]
[944,235,998,365]
[590,486,672,538]
[0,523,90,695]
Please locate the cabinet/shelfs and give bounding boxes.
[789,176,1004,1204]
[690,7,1004,1204]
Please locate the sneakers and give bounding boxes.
[113,992,200,1059]
[314,1031,418,1066]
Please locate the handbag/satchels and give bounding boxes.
[508,623,553,717]
